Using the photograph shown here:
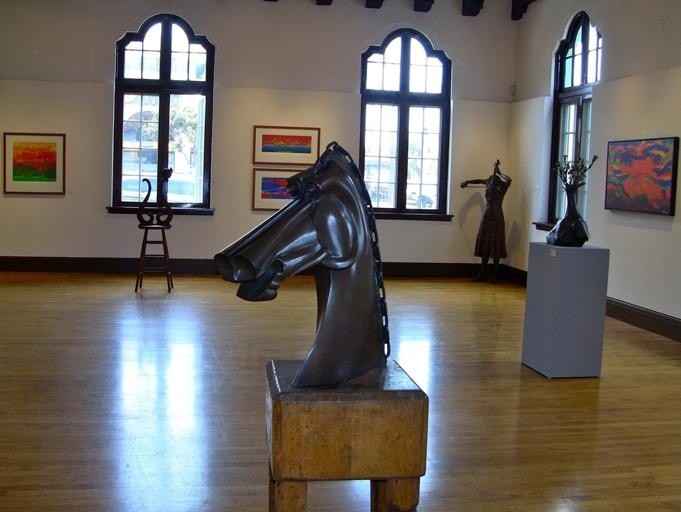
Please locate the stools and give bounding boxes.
[135,224,173,293]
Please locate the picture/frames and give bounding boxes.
[252,168,306,211]
[605,137,679,216]
[4,132,65,195]
[253,126,320,166]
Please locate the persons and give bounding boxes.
[460,159,512,284]
